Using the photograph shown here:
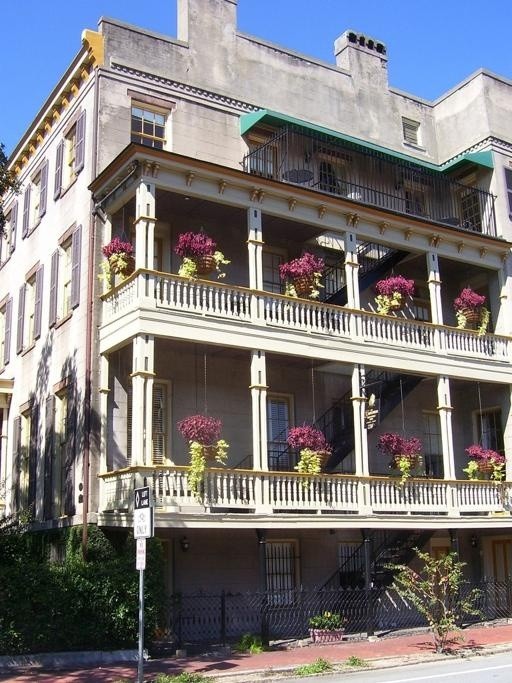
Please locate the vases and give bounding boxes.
[313,449,331,472]
[309,625,345,643]
[200,443,218,460]
[293,274,313,293]
[477,460,495,473]
[387,296,406,311]
[463,305,481,324]
[393,453,418,470]
[109,254,134,274]
[190,253,217,275]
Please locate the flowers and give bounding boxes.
[95,237,133,290]
[280,250,326,299]
[307,609,344,629]
[177,413,230,495]
[286,422,334,486]
[374,275,416,314]
[463,444,508,481]
[454,285,492,335]
[171,230,231,281]
[376,430,423,491]
